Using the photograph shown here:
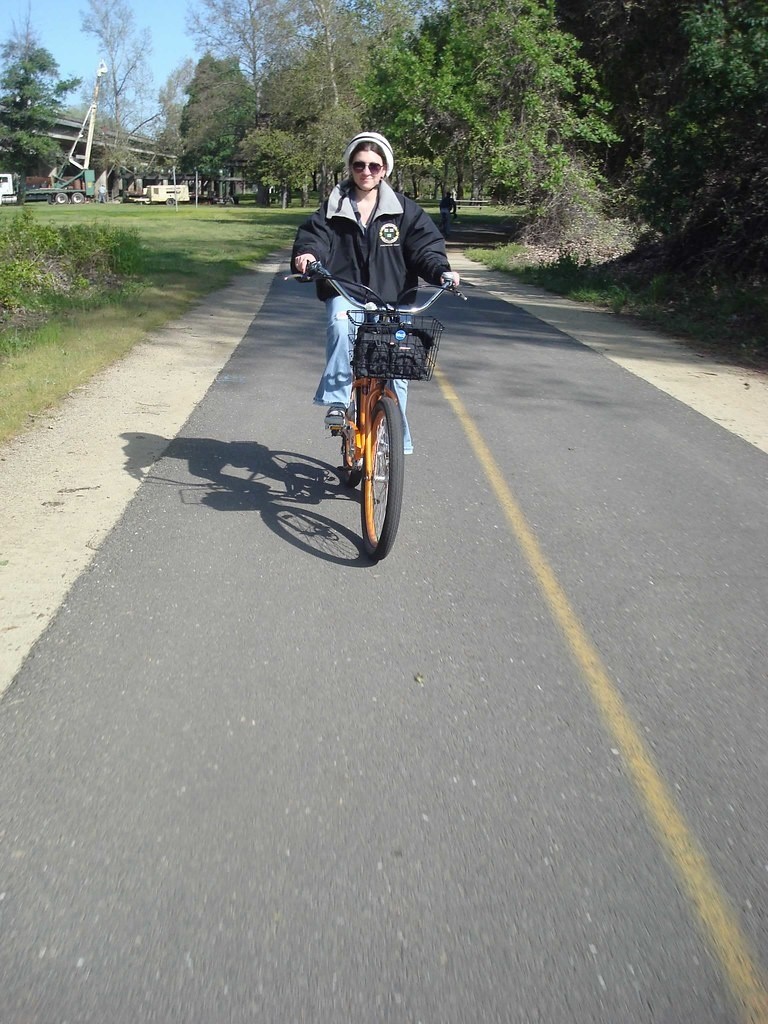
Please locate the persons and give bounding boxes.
[98,185,106,202]
[290,131,460,456]
[439,192,456,235]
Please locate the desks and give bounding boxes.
[455,200,488,210]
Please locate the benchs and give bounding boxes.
[456,205,488,208]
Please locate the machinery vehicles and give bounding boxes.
[0,58,108,206]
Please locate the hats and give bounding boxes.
[343,131,394,181]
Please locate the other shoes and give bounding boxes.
[324,405,346,425]
[374,451,389,482]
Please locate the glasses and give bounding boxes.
[349,161,387,175]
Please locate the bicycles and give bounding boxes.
[284,260,467,560]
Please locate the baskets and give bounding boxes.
[346,310,446,381]
